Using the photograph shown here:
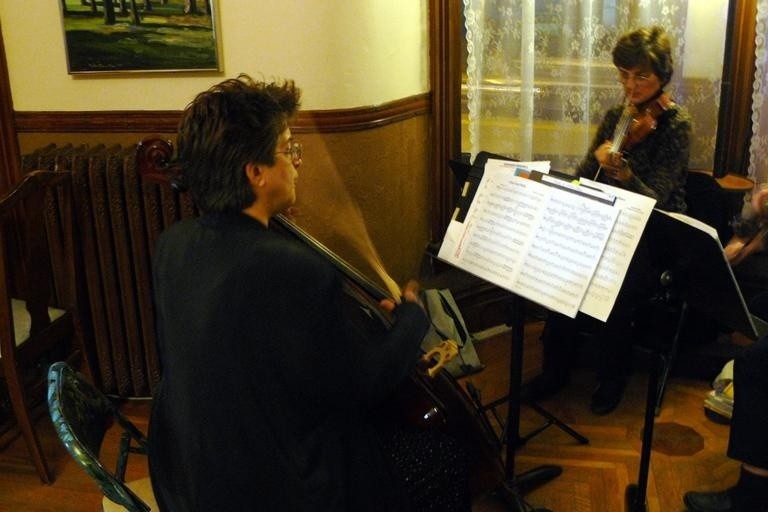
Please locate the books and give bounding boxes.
[443,154,657,328]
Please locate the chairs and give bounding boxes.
[0,167,102,486]
[43,357,151,512]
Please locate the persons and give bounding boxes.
[718,184,768,322]
[532,25,691,421]
[684,331,768,512]
[141,71,499,512]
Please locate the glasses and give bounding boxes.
[612,68,652,87]
[272,141,305,163]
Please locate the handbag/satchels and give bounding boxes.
[418,287,485,382]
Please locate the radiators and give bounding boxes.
[17,140,200,403]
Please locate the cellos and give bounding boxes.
[134,136,501,474]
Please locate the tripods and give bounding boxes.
[478,298,588,489]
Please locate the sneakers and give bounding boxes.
[588,364,628,415]
[520,366,571,404]
[683,483,768,510]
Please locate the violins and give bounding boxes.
[604,93,674,166]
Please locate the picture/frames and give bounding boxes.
[55,0,224,77]
